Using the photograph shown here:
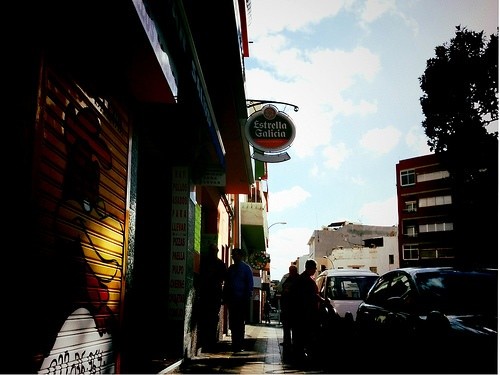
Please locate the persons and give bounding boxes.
[198,243,253,351]
[264,299,271,321]
[280,260,334,347]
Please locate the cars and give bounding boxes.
[316,267,381,323]
[356,266,498,346]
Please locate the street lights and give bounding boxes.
[323,256,335,269]
[268,221,287,229]
